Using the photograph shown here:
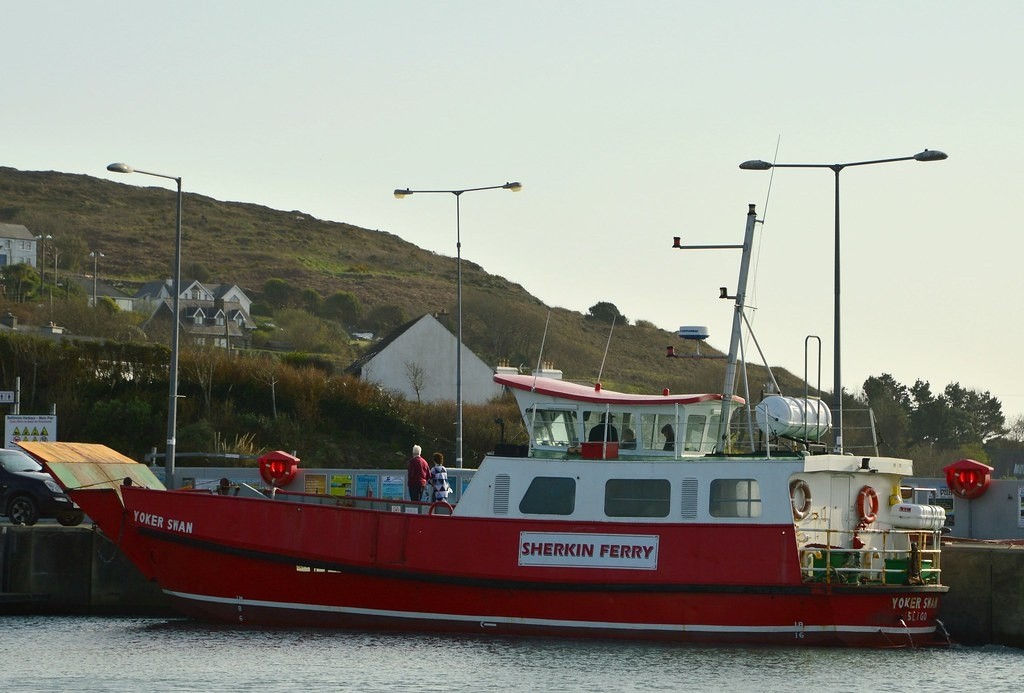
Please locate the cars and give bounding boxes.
[0,448,84,526]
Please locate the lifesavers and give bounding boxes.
[791,479,811,519]
[857,486,879,524]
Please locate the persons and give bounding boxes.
[588,412,619,446]
[429,453,453,502]
[661,424,675,451]
[618,428,637,450]
[407,445,432,501]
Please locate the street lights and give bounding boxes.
[107,161,183,492]
[394,181,522,504]
[739,148,948,455]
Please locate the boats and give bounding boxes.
[13,205,955,652]
